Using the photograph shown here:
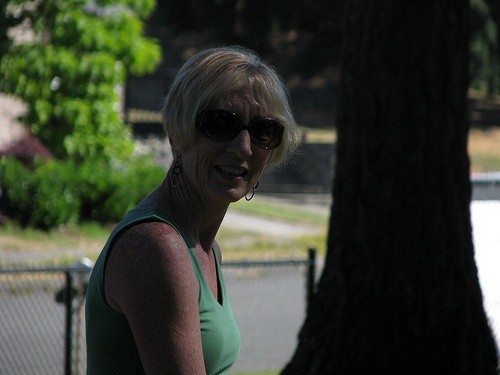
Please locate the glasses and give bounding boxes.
[195,108,285,150]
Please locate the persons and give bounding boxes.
[85,48,301,375]
